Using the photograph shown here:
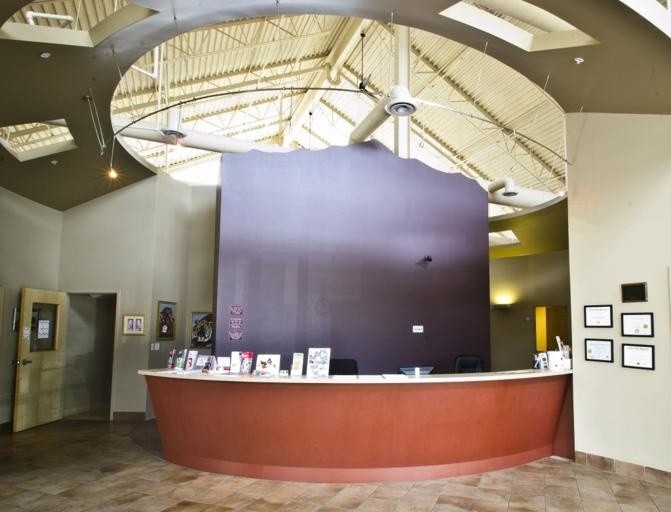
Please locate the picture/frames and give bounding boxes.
[189,311,215,348]
[584,305,613,328]
[621,312,654,337]
[157,300,177,341]
[12,307,39,331]
[122,313,146,336]
[584,338,613,364]
[621,344,655,371]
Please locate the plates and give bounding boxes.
[400,366,434,375]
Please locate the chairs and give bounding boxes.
[454,355,484,373]
[329,358,359,375]
